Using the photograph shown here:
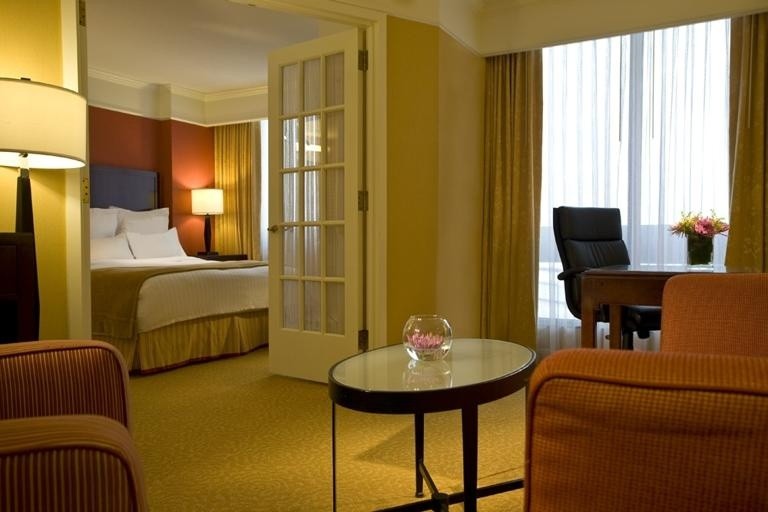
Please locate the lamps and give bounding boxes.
[552,207,662,349]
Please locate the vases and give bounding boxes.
[668,209,730,235]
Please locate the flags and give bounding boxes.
[684,236,713,266]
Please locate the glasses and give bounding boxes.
[0,75,87,338]
[191,187,226,255]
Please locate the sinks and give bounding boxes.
[527,273,764,511]
[1,341,149,512]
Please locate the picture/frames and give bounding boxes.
[580,264,762,347]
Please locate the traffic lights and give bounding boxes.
[90,208,119,234]
[125,229,184,255]
[113,206,170,231]
[87,234,130,260]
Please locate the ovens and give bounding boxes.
[90,164,267,376]
